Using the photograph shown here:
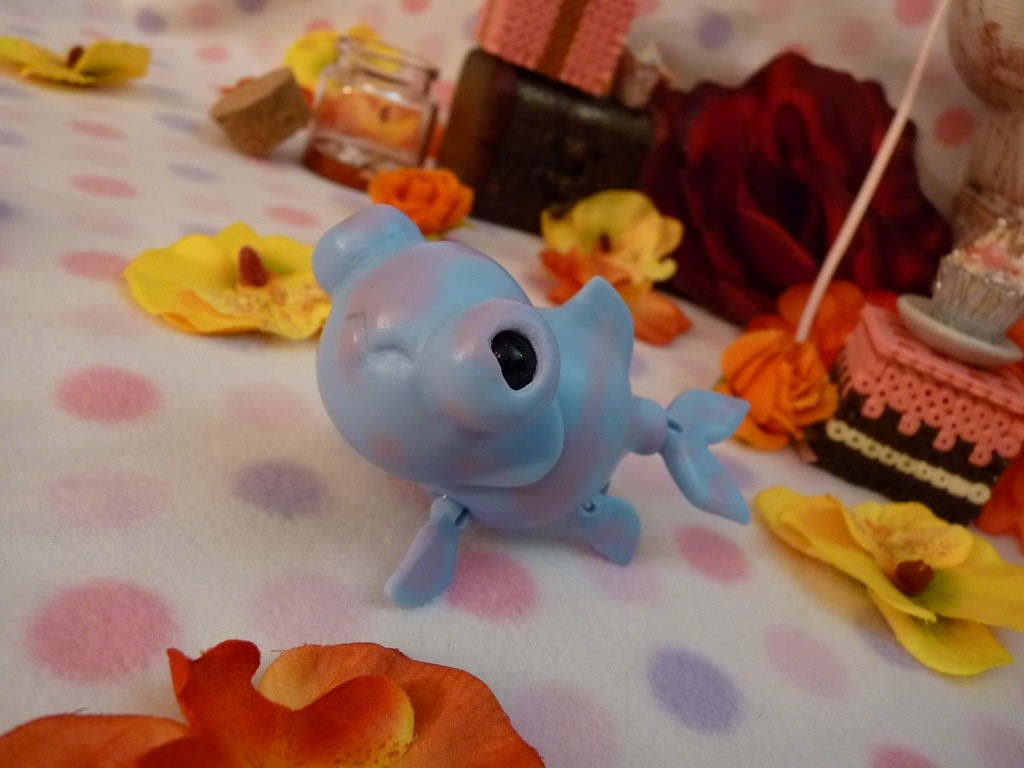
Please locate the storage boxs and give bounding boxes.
[803,302,1024,529]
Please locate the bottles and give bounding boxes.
[299,36,440,192]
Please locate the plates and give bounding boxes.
[897,294,1023,368]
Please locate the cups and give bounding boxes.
[929,256,1023,343]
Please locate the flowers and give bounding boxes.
[754,486,1024,677]
[369,169,483,248]
[121,220,335,342]
[0,637,548,768]
[711,280,904,465]
[540,191,696,347]
